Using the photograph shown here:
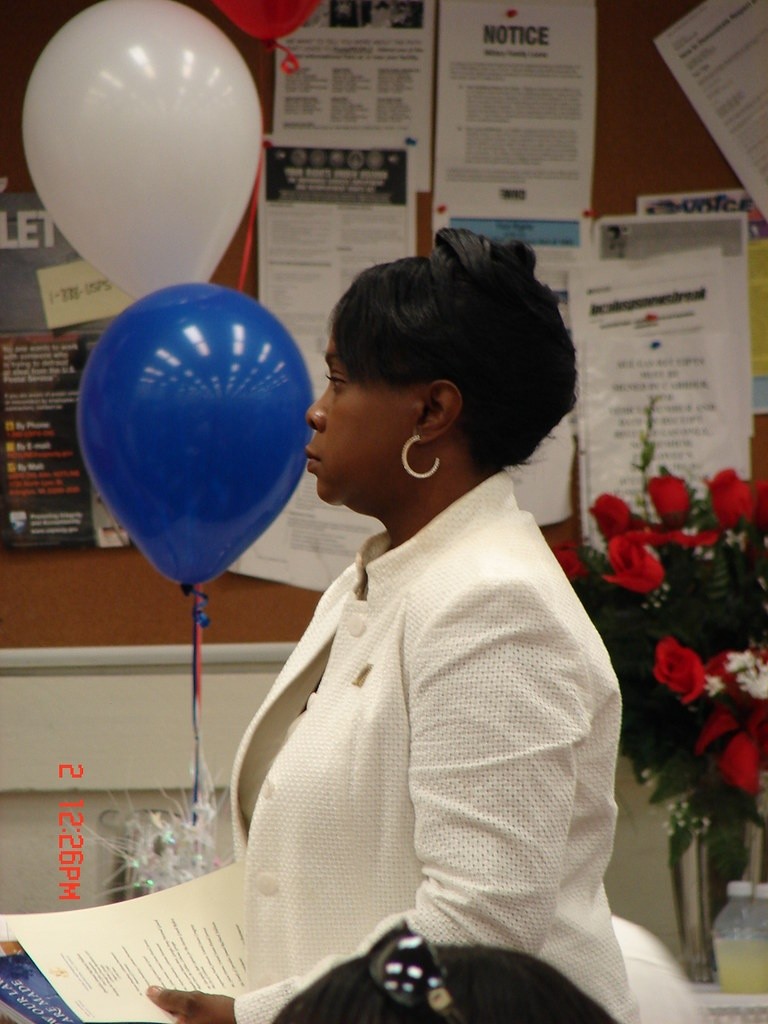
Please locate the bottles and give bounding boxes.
[710,882,767,994]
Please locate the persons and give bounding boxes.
[146,225,640,1024]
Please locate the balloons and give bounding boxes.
[215,0,320,40]
[77,283,314,594]
[22,0,262,300]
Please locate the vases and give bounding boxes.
[669,821,746,982]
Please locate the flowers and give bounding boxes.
[553,393,768,878]
